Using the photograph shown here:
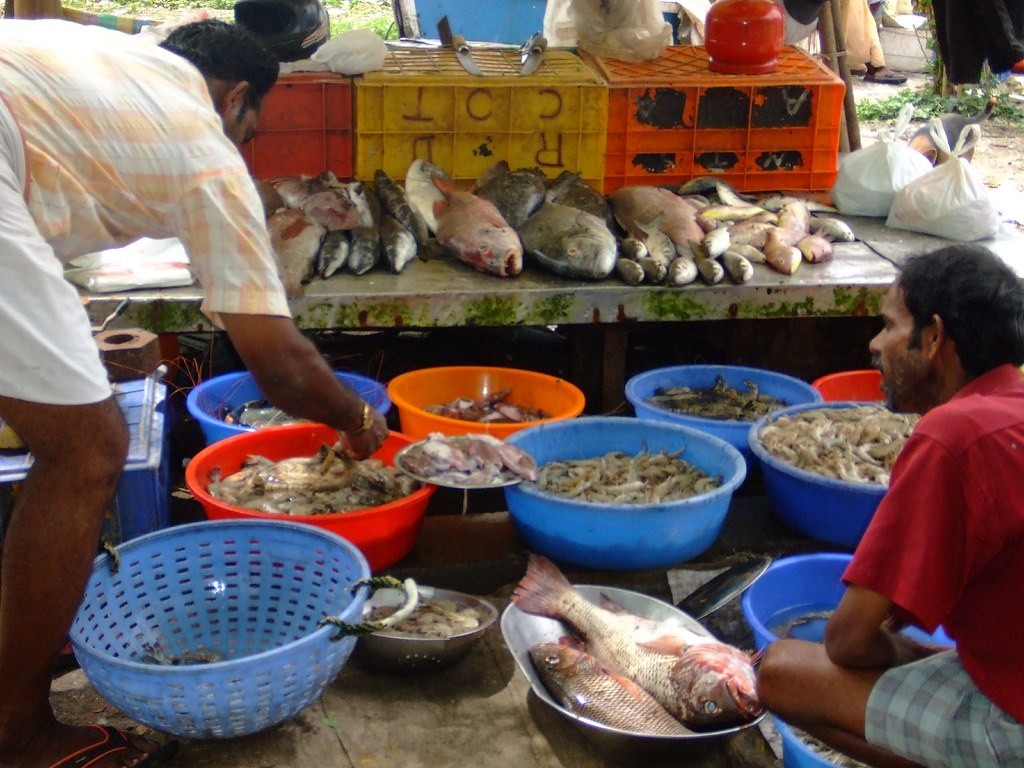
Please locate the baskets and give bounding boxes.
[66,519,422,738]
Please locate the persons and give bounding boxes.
[860,0,906,83]
[759,247,1024,768]
[1,17,388,768]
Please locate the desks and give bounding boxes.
[82,210,1024,417]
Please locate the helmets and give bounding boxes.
[231,0,331,62]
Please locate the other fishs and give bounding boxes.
[510,553,761,731]
[402,431,538,486]
[269,156,856,301]
[527,641,700,736]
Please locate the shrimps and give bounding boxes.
[645,378,788,421]
[530,442,724,505]
[758,401,921,486]
[206,439,421,516]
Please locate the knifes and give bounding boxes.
[452,35,484,77]
[520,37,547,76]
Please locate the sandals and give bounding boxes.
[863,66,907,85]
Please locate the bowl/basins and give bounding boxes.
[747,402,911,549]
[498,416,748,571]
[741,552,957,660]
[386,366,585,446]
[185,424,438,573]
[811,370,887,403]
[500,584,770,768]
[772,715,873,768]
[625,364,826,488]
[187,370,392,447]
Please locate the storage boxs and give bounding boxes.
[0,365,171,552]
[577,43,847,197]
[353,47,608,197]
[237,73,352,184]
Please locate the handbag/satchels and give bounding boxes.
[885,116,998,242]
[829,101,936,216]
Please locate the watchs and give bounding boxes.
[347,401,374,435]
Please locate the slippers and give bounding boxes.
[49,724,180,768]
